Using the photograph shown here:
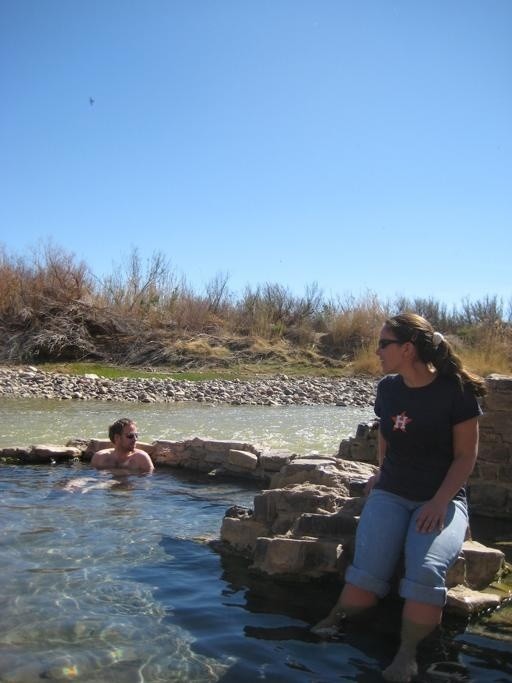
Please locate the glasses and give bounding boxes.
[122,433,137,438]
[378,338,399,349]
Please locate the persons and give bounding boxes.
[309,312,487,682]
[63,417,144,495]
